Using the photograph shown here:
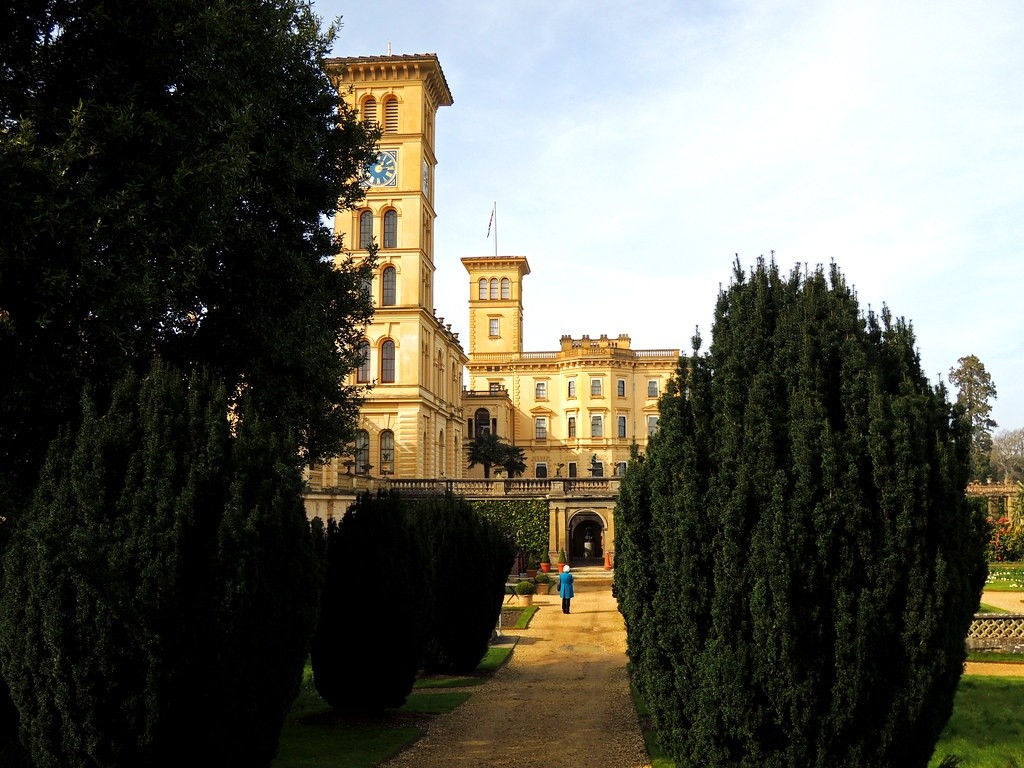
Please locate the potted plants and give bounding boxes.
[516,581,535,605]
[526,553,537,577]
[539,545,551,573]
[556,548,569,573]
[534,574,549,595]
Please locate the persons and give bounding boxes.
[559,565,574,614]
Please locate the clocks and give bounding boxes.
[358,150,397,187]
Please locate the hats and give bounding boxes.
[562,565,570,572]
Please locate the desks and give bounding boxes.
[505,574,534,604]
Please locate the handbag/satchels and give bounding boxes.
[557,583,561,591]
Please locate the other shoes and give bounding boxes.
[563,610,570,614]
